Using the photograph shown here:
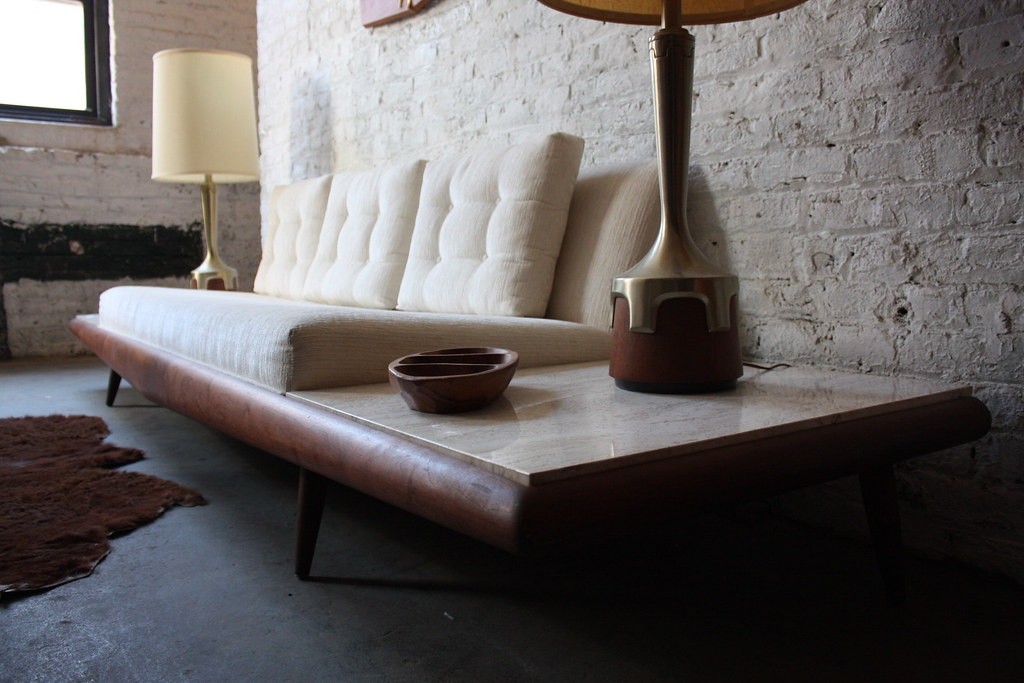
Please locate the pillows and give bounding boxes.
[253,132,586,318]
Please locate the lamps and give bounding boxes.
[537,0,808,396]
[150,48,260,291]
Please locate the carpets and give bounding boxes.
[0,415,208,593]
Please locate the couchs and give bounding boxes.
[69,131,993,580]
